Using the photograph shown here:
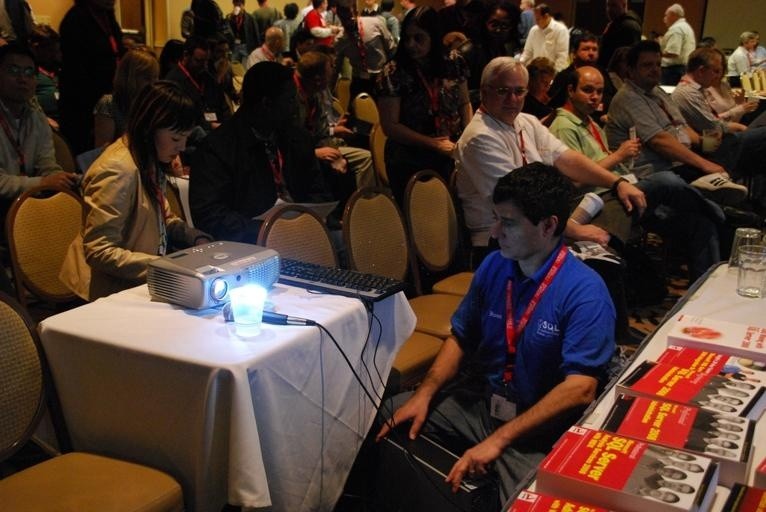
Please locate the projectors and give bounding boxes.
[146,241,281,310]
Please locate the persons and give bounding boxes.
[331,158,619,511]
[1,0,761,348]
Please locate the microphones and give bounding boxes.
[222,302,316,325]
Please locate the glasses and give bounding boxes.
[491,85,529,98]
[3,62,37,78]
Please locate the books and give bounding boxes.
[502,311,764,511]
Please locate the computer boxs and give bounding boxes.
[380,429,500,512]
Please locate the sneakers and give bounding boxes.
[691,172,747,202]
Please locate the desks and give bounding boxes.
[496,260,766,512]
[39,267,418,512]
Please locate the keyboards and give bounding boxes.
[279,256,409,302]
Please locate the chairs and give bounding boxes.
[255,206,444,480]
[6,183,88,308]
[407,170,478,298]
[740,66,766,104]
[343,187,466,338]
[0,296,184,511]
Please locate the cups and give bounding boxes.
[571,191,604,226]
[737,244,766,299]
[702,130,721,155]
[748,92,761,112]
[728,228,763,278]
[227,284,267,340]
